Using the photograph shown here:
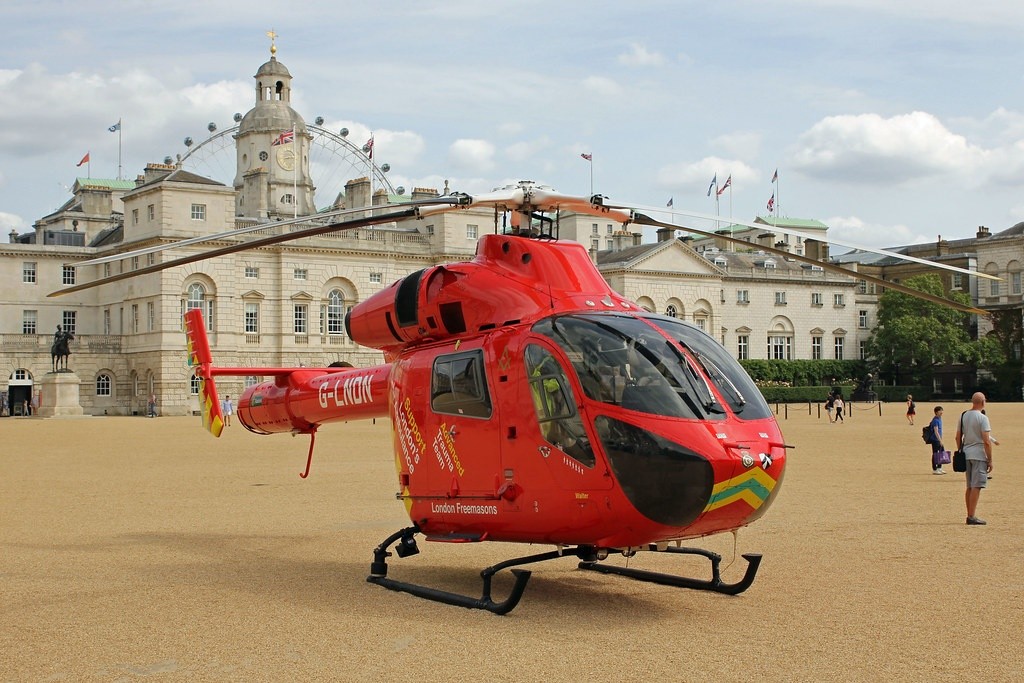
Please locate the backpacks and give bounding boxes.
[922,417,939,444]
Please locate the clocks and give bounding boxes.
[276,145,299,170]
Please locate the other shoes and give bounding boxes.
[967,517,986,525]
[937,468,946,474]
[933,470,942,475]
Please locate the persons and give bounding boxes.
[906,395,914,425]
[826,392,835,423]
[31,394,37,415]
[981,410,999,479]
[54,324,72,355]
[930,406,946,475]
[955,392,993,525]
[24,398,29,416]
[834,395,844,422]
[4,397,9,415]
[151,392,158,417]
[222,395,233,426]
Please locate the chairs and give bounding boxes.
[432,393,489,422]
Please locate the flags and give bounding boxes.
[667,197,673,206]
[369,136,374,159]
[581,153,592,160]
[272,125,295,145]
[707,175,716,196]
[76,152,90,166]
[772,169,777,182]
[109,119,121,132]
[767,193,774,212]
[717,174,731,194]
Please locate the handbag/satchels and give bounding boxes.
[910,404,915,410]
[953,451,966,472]
[825,404,830,409]
[934,446,951,464]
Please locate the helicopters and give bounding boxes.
[47,178,1003,616]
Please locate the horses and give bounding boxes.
[51,332,76,372]
